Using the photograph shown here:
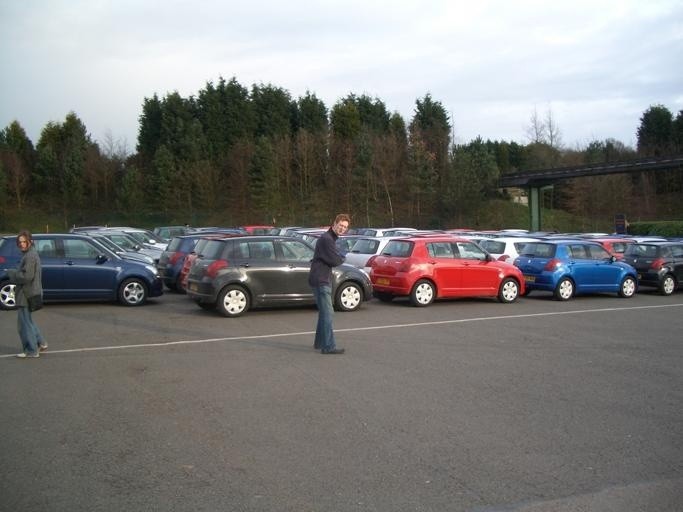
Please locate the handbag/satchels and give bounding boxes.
[27,294,43,312]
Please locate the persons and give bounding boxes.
[3,230,48,358]
[308,214,351,354]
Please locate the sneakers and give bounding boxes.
[314,344,344,354]
[16,343,48,358]
